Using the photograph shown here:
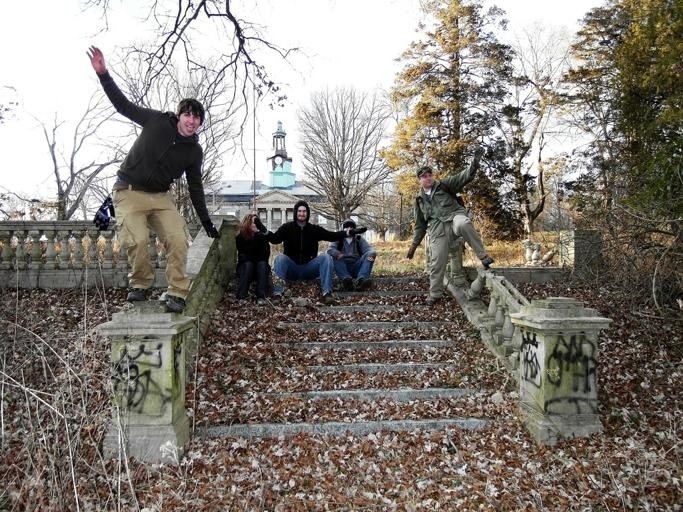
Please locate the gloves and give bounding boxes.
[346,226,369,237]
[474,146,485,165]
[252,216,268,234]
[405,244,417,260]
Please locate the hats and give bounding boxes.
[415,166,432,176]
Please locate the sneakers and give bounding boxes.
[479,253,494,267]
[425,296,441,305]
[165,295,185,313]
[236,278,374,307]
[127,285,153,303]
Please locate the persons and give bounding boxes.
[232,199,376,309]
[85,45,222,314]
[406,146,494,306]
